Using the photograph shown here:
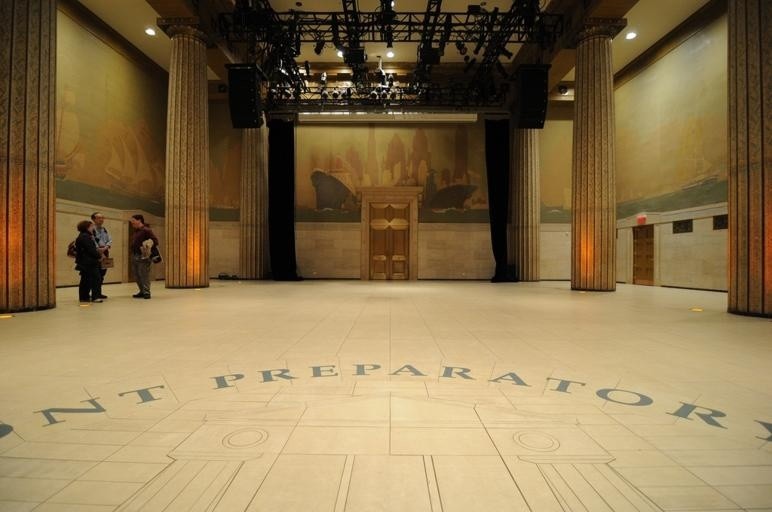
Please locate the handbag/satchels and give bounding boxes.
[100,257,114,269]
[66,241,76,258]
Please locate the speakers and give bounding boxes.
[515,63,552,129]
[224,62,264,129]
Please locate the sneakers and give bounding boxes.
[79,294,108,303]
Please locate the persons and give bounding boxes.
[74,219,103,301]
[90,211,113,299]
[128,214,159,299]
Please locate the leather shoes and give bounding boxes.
[132,291,150,300]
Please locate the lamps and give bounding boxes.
[313,31,326,55]
[271,87,397,106]
[386,25,395,48]
